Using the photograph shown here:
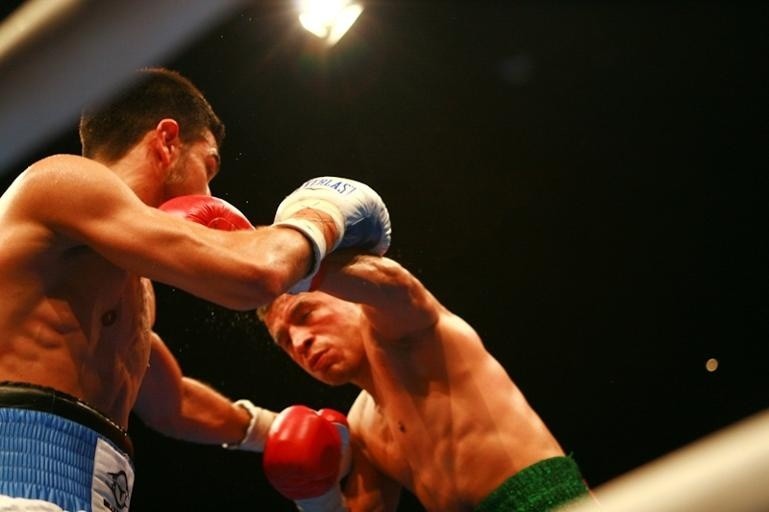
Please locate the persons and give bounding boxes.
[0,63,391,512]
[157,193,600,512]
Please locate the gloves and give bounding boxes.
[268,176,391,281]
[225,398,353,481]
[264,405,347,511]
[158,194,253,234]
